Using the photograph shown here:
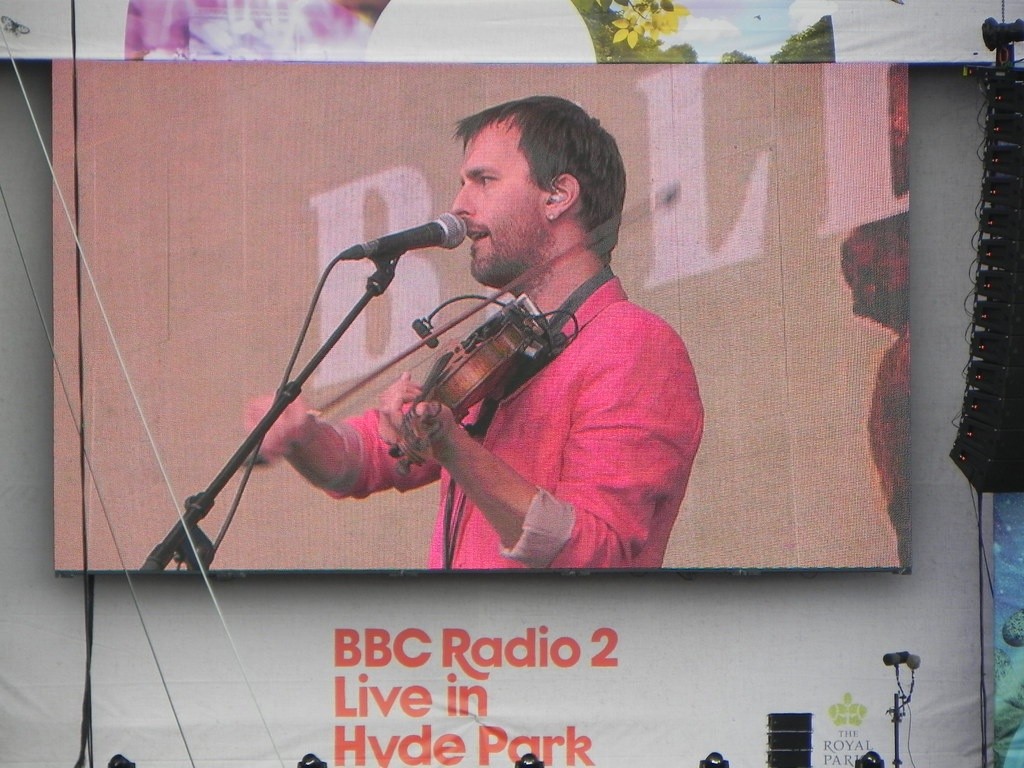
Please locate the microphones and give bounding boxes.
[883,651,921,669]
[338,212,467,262]
[411,294,527,348]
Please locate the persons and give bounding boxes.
[242,94,705,570]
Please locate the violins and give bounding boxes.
[386,293,566,476]
